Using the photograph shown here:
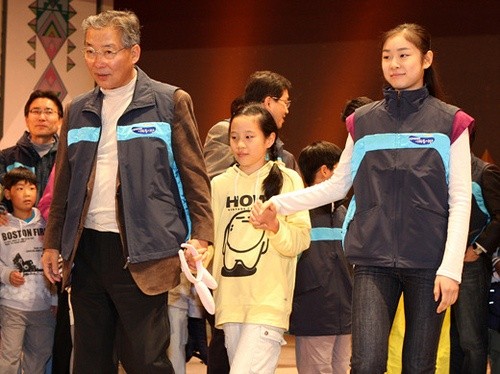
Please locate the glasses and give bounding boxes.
[272,96,291,108]
[81,46,125,58]
[28,107,59,114]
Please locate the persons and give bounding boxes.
[229,94,250,118]
[0,165,59,374]
[288,141,355,374]
[0,89,65,228]
[178,104,313,374]
[486,240,500,374]
[453,124,500,374]
[341,96,374,124]
[252,23,476,374]
[42,10,215,374]
[203,71,304,374]
[167,269,192,374]
